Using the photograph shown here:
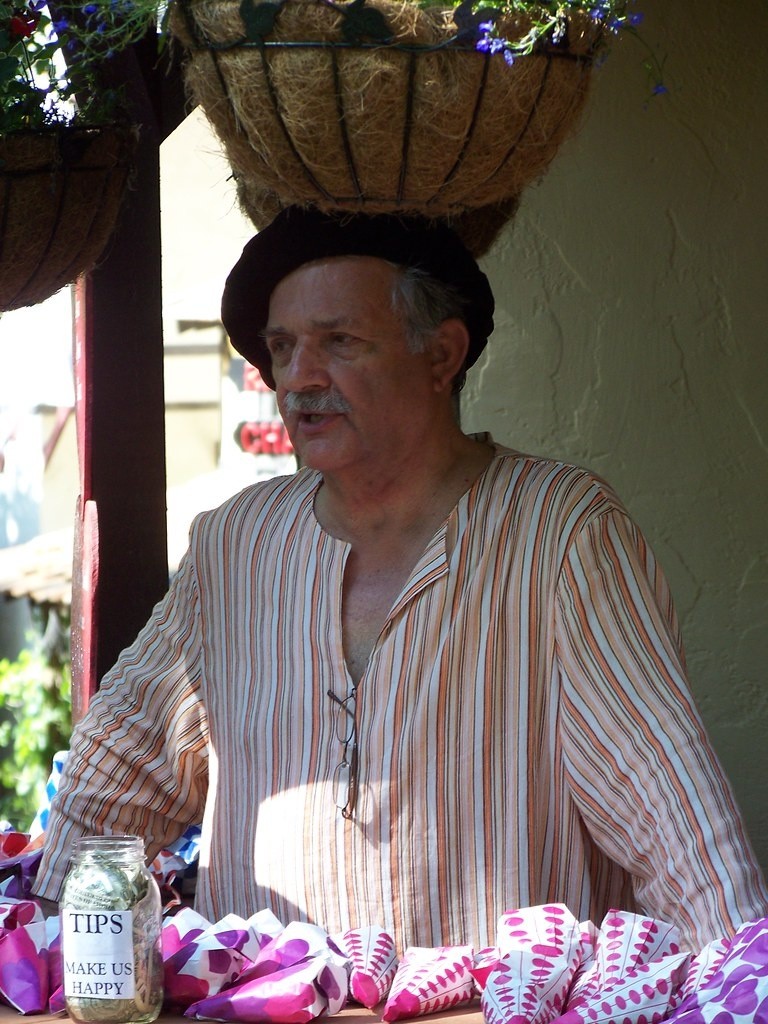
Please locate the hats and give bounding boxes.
[221,203,494,368]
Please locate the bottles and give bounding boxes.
[59,834,164,1024]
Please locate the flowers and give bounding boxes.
[0,0,671,313]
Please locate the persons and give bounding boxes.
[18,203,767,961]
